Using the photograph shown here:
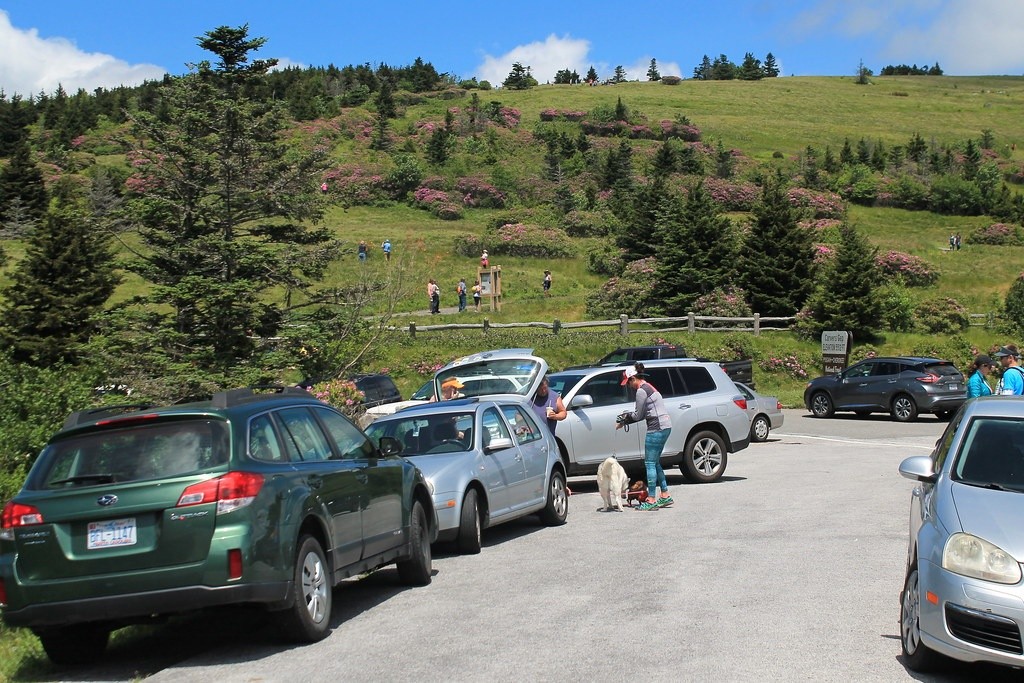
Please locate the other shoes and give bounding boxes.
[634,500,659,511]
[657,496,673,507]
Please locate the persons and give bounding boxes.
[481,249,488,269]
[428,376,465,445]
[458,276,466,312]
[542,270,552,297]
[546,78,612,87]
[358,240,368,264]
[472,280,481,312]
[994,345,1024,396]
[948,232,961,250]
[1011,143,1016,150]
[514,375,567,438]
[495,85,498,90]
[966,355,994,400]
[381,239,391,261]
[428,279,441,314]
[321,182,327,195]
[615,363,673,511]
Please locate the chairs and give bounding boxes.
[464,427,491,451]
[402,427,429,455]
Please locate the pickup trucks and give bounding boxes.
[564,344,756,392]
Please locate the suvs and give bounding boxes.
[0,384,440,669]
[349,371,404,411]
[804,357,967,422]
[362,374,547,422]
[523,358,750,483]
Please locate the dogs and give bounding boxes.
[596,457,630,512]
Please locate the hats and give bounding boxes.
[441,379,464,390]
[975,355,996,365]
[620,366,649,385]
[544,270,551,274]
[994,346,1019,356]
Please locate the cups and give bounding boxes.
[546,407,554,417]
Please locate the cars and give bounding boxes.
[733,381,784,441]
[898,393,1024,675]
[341,348,568,555]
